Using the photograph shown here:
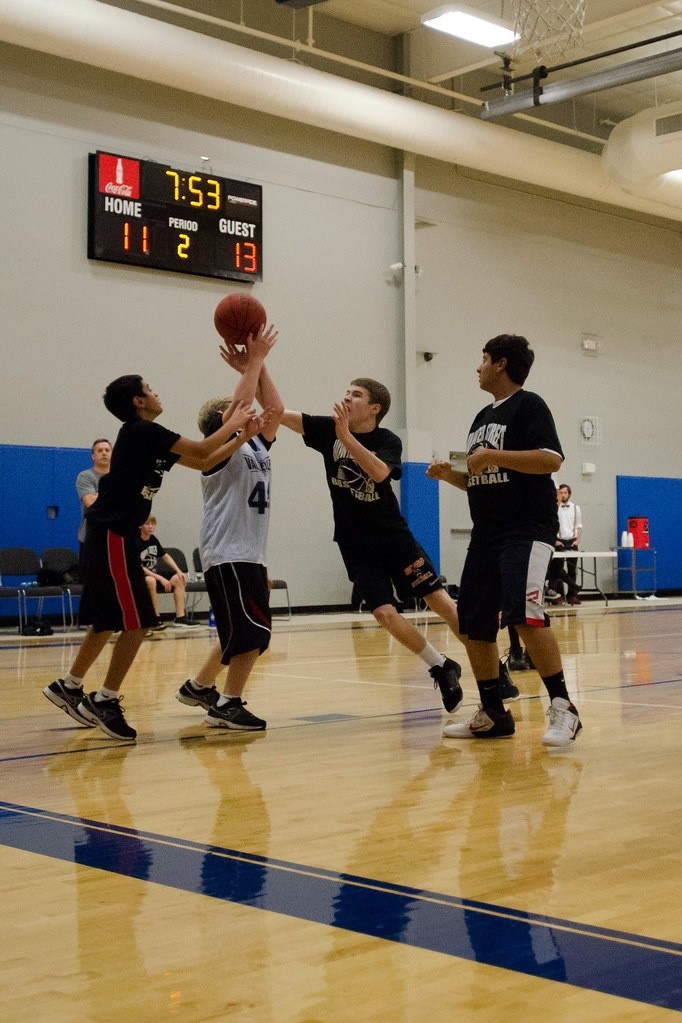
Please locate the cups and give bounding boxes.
[621,530,634,548]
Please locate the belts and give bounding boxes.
[561,539,573,542]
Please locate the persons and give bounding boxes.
[425,334,581,746]
[175,323,284,731]
[41,374,276,739]
[216,339,521,714]
[76,438,199,635]
[500,485,583,671]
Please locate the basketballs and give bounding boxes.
[213,291,267,345]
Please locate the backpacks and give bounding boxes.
[22,615,53,635]
[36,566,68,587]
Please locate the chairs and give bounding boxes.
[0,547,448,634]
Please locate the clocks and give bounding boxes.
[581,420,595,440]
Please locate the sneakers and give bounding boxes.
[499,658,519,701]
[508,647,537,672]
[427,653,464,713]
[542,697,583,748]
[172,615,201,628]
[553,594,580,605]
[42,678,137,740]
[154,616,163,626]
[109,625,166,642]
[175,679,267,731]
[442,705,516,739]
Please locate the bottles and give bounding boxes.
[20,581,41,588]
[209,609,217,630]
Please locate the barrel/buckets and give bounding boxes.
[627,517,650,549]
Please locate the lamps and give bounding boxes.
[420,2,525,48]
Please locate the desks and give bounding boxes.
[553,551,617,606]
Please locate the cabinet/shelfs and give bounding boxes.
[610,546,657,599]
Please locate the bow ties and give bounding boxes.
[561,504,570,508]
